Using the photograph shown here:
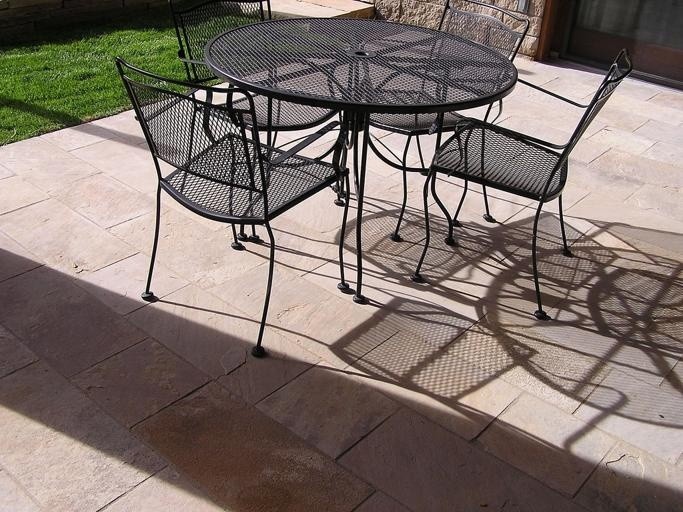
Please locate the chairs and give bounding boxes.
[335,0,531,243]
[114,56,350,357]
[168,0,349,242]
[410,48,633,320]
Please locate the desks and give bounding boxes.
[206,18,519,292]
